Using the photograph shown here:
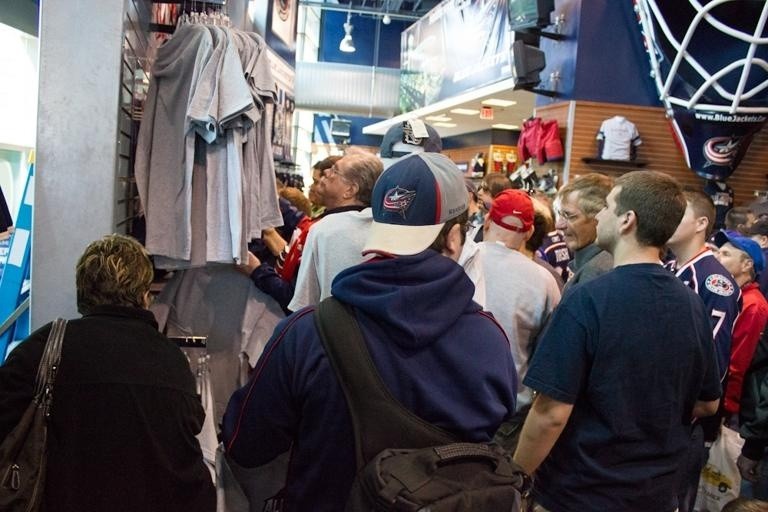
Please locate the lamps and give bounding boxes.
[338,1,356,53]
[382,1,391,24]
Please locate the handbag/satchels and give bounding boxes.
[0,317,69,512]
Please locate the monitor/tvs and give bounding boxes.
[505,1,556,34]
[512,39,546,82]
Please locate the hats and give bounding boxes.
[465,180,478,196]
[713,227,766,274]
[489,188,535,234]
[380,118,443,158]
[362,150,471,262]
[743,220,768,237]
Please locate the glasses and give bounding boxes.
[556,206,584,222]
[460,220,477,233]
[477,184,492,195]
[324,163,350,182]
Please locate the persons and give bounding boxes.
[275,156,347,282]
[705,230,729,254]
[520,162,538,192]
[714,230,768,433]
[507,150,518,174]
[262,160,324,257]
[234,153,384,317]
[664,190,744,510]
[215,152,518,511]
[738,220,768,302]
[287,117,487,316]
[471,152,488,179]
[531,194,574,283]
[511,169,724,512]
[251,187,312,263]
[467,173,511,242]
[751,201,768,220]
[493,150,504,173]
[0,233,218,512]
[596,115,643,163]
[703,181,735,231]
[519,197,565,294]
[516,173,619,364]
[458,190,564,431]
[725,207,756,236]
[737,319,768,503]
[465,183,479,226]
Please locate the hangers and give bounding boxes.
[174,0,231,28]
[180,335,212,379]
